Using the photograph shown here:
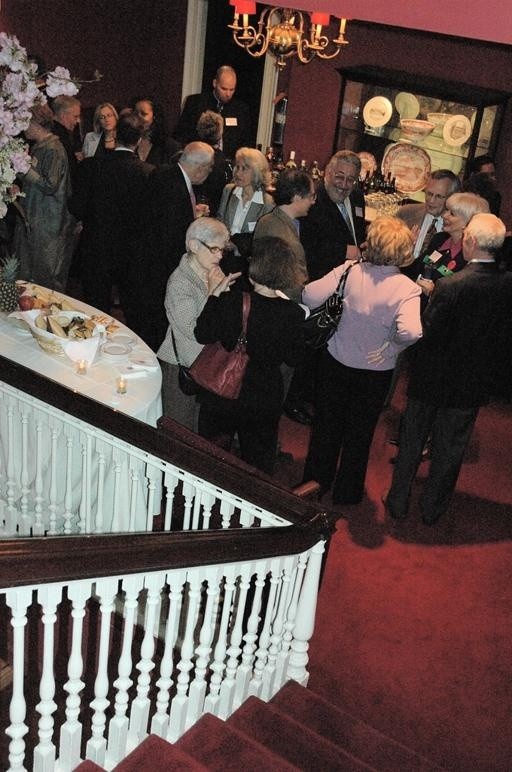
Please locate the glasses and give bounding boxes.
[424,191,447,200]
[201,242,224,254]
[330,172,357,185]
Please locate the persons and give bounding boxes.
[49,95,83,178]
[217,146,275,336]
[284,149,366,425]
[133,98,181,165]
[143,141,210,271]
[173,64,251,156]
[157,217,231,430]
[196,110,232,204]
[382,213,512,524]
[73,113,152,314]
[394,170,459,229]
[193,235,305,470]
[300,214,422,504]
[9,104,77,296]
[254,168,317,288]
[417,191,490,312]
[472,154,502,213]
[82,102,118,159]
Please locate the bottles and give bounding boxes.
[286,151,298,169]
[258,144,262,150]
[300,160,306,171]
[363,170,370,195]
[372,169,397,193]
[274,151,284,172]
[266,147,273,172]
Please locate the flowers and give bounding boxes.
[2,30,102,231]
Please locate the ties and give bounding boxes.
[420,219,438,256]
[337,201,353,236]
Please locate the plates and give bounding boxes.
[108,331,133,344]
[100,343,133,361]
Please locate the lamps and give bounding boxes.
[226,0,352,70]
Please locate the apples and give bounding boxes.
[19,295,35,311]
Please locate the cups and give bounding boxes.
[115,376,127,392]
[74,359,86,375]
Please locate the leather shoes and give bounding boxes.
[388,433,431,445]
[381,489,407,519]
[388,447,429,467]
[285,405,313,428]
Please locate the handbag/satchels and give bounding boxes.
[188,293,251,400]
[170,331,197,396]
[301,261,358,350]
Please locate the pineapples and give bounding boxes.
[1,257,19,312]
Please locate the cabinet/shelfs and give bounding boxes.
[330,61,510,216]
